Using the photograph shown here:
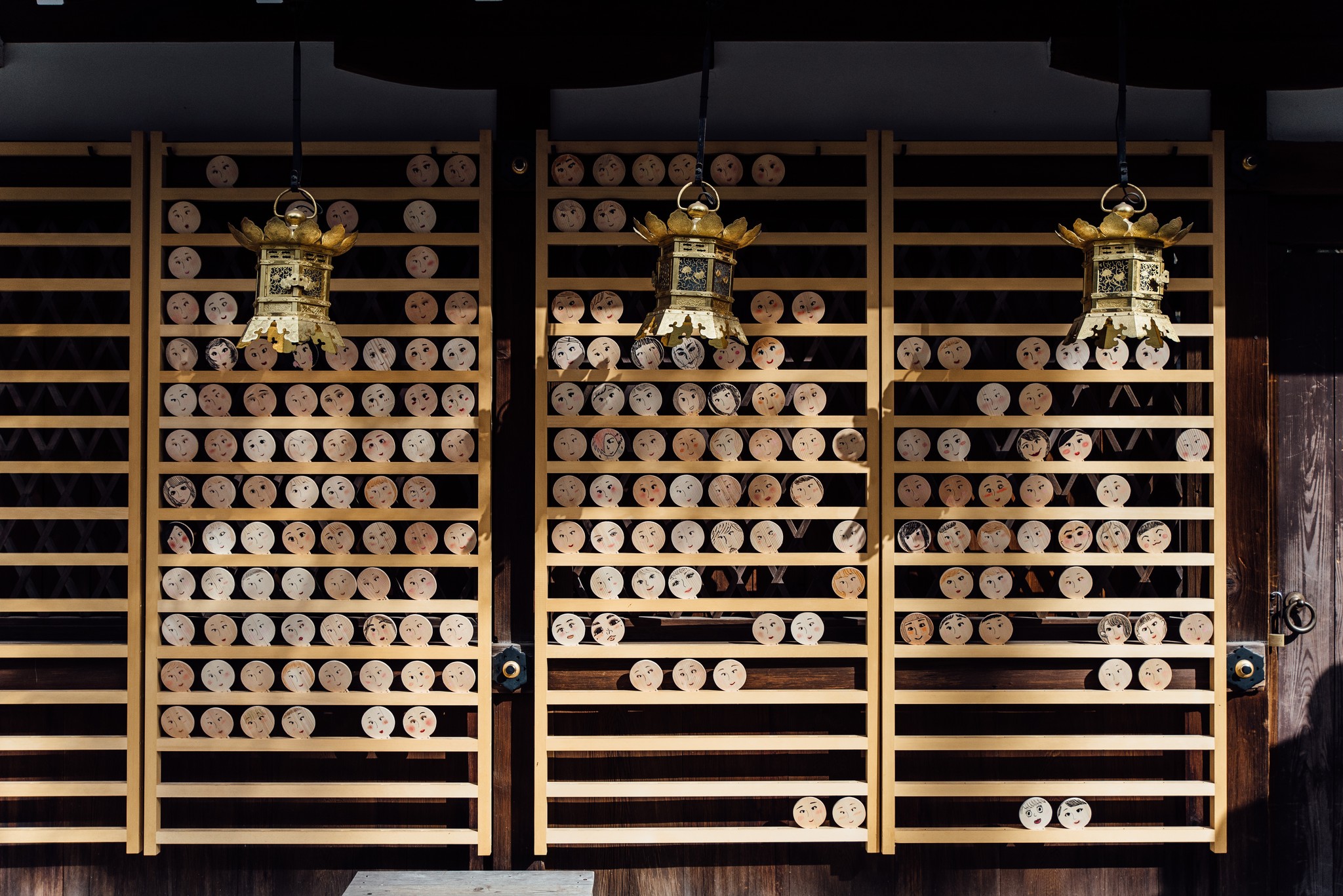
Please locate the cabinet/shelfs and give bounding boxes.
[0,118,1230,856]
